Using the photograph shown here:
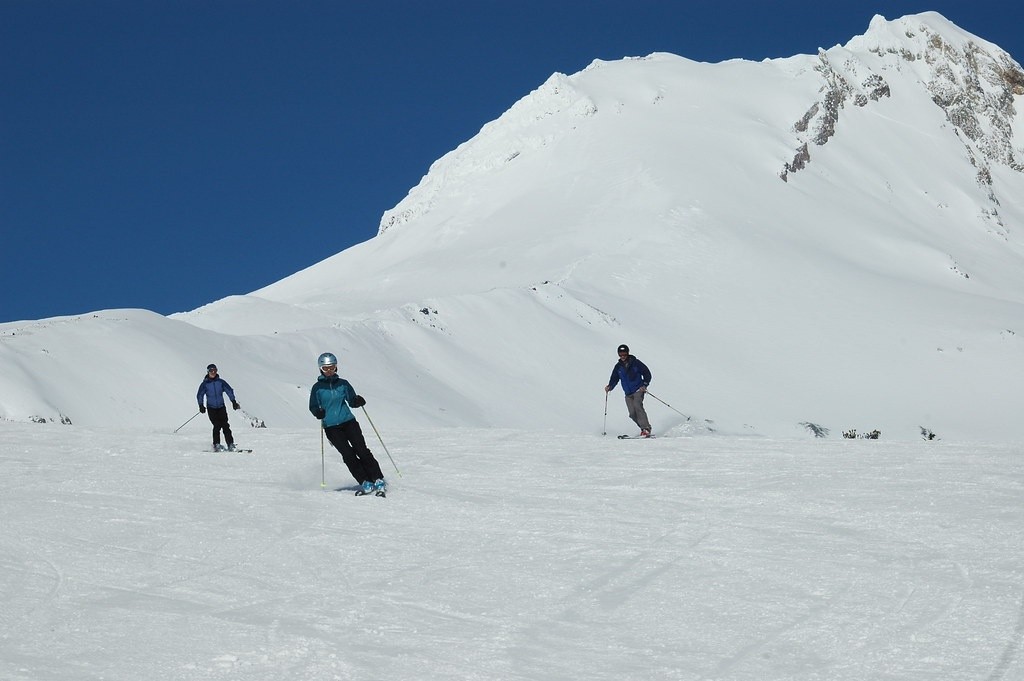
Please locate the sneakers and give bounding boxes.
[355,479,386,497]
[213,443,237,452]
[641,428,650,437]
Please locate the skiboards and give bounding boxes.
[353,483,388,497]
[617,434,660,440]
[206,447,255,454]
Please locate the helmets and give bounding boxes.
[318,352,338,365]
[617,345,629,352]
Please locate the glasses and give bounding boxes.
[319,365,337,372]
[207,369,218,372]
[619,354,628,357]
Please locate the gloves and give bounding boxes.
[314,408,325,419]
[640,388,645,394]
[605,386,611,392]
[353,395,366,407]
[199,405,206,413]
[231,400,239,410]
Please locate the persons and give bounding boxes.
[197,364,243,453]
[309,352,388,497]
[605,344,652,437]
